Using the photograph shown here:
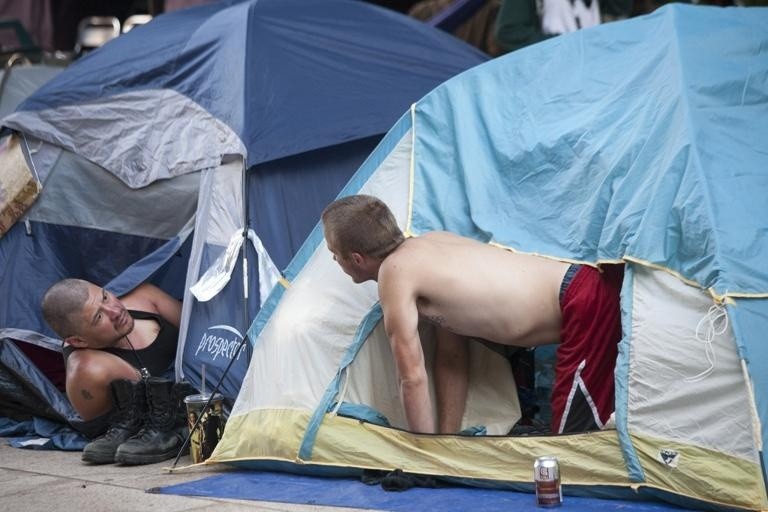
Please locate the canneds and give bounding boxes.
[533,455,563,507]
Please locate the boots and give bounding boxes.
[114,376,196,464]
[81,377,147,463]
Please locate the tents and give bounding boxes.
[0,0,494,451]
[203,0,768,512]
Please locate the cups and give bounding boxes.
[184,393,225,465]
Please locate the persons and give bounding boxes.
[189,405,227,463]
[321,195,623,438]
[39,278,183,421]
